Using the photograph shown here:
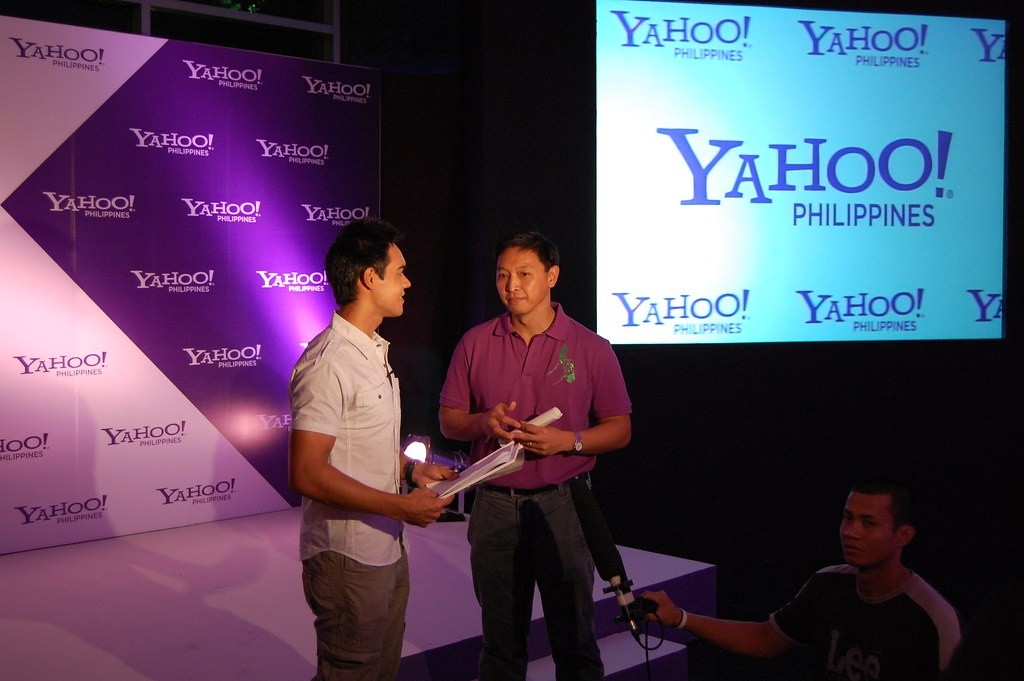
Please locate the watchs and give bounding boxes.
[572,432,582,455]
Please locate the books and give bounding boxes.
[426,441,520,498]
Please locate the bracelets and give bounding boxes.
[405,460,421,488]
[672,608,687,630]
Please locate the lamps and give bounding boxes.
[400,434,433,466]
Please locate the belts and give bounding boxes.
[483,473,588,495]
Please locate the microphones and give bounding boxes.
[570,477,641,643]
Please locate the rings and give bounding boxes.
[529,442,532,448]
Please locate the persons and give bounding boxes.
[439,231,631,681]
[643,474,968,681]
[288,219,460,681]
